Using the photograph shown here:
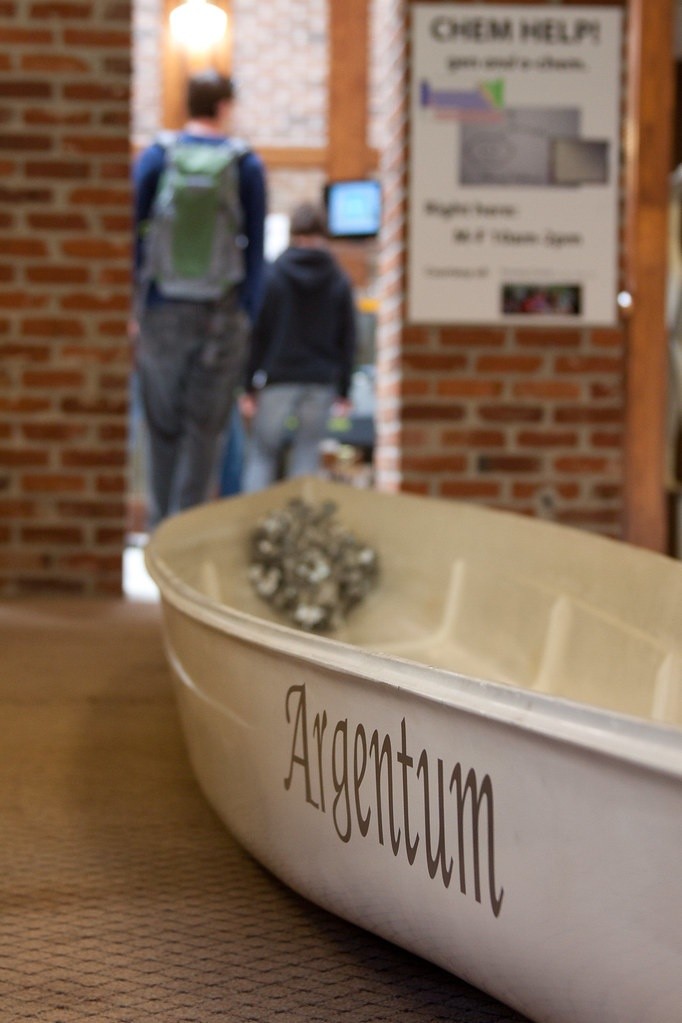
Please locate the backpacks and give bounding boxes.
[130,132,253,329]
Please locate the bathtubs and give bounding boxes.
[143,481,682,1022]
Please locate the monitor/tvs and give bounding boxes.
[324,181,382,240]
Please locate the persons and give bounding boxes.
[236,202,356,497]
[218,386,255,500]
[129,71,270,530]
